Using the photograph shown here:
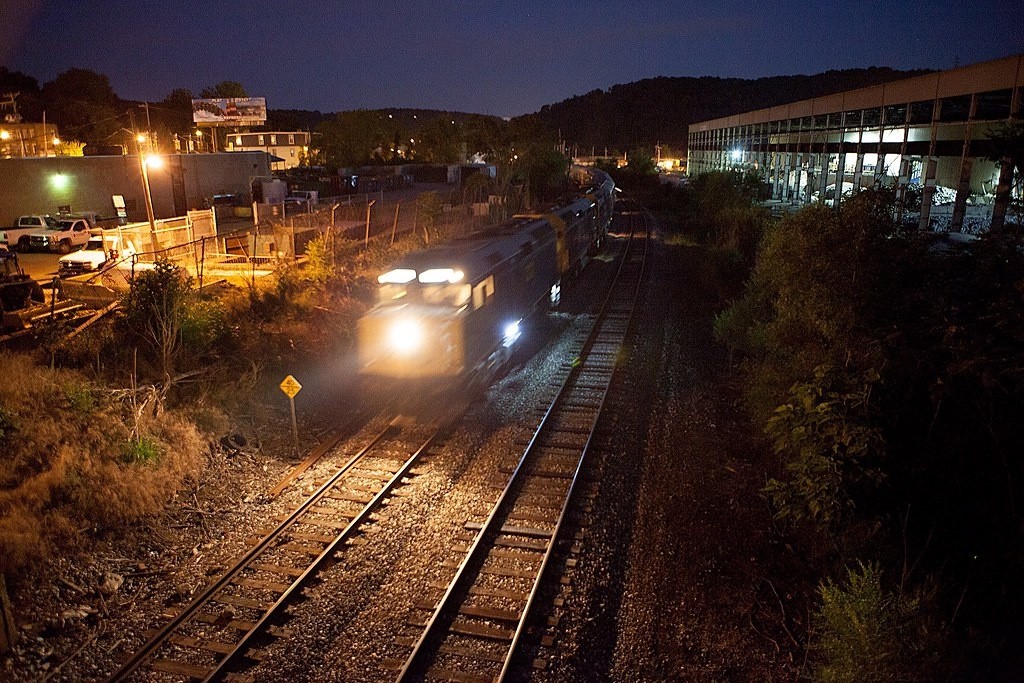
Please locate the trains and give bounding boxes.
[355,163,618,392]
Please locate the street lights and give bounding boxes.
[139,154,163,262]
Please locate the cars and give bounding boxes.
[57,234,139,274]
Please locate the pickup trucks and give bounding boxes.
[0,215,107,254]
